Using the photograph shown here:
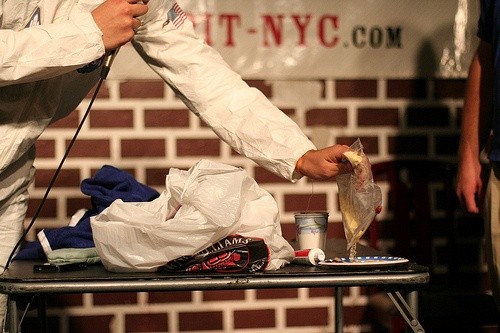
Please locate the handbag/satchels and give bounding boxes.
[89,159,296,271]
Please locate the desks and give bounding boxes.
[0,239,429,333]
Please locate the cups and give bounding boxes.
[294,211,328,253]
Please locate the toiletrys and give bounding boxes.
[293,248,325,266]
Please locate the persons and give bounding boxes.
[456,0,500,316]
[1,0,369,333]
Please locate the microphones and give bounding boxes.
[100,0,146,80]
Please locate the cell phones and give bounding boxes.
[33,261,87,273]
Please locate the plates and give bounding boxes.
[317,254,410,268]
